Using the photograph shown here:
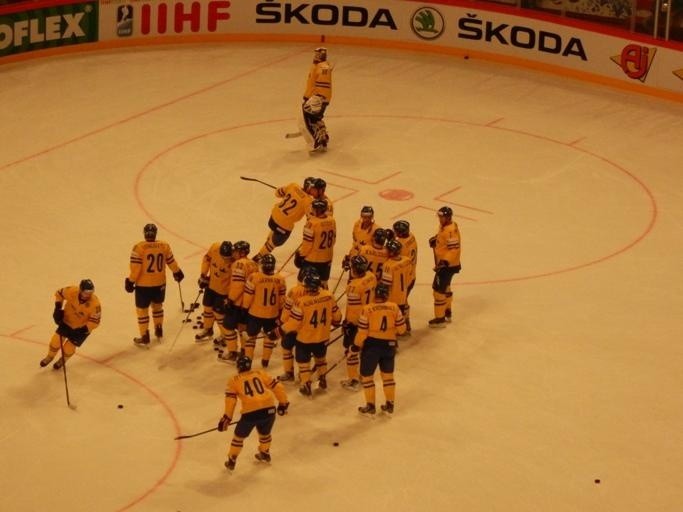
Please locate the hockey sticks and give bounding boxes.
[319,353,347,389]
[56,323,76,410]
[175,422,239,440]
[285,130,303,138]
[240,176,276,189]
[158,289,202,369]
[177,281,185,312]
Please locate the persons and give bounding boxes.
[40,278,101,369]
[341,205,417,415]
[429,207,461,325]
[219,356,290,470]
[303,47,332,148]
[125,224,184,343]
[197,177,342,394]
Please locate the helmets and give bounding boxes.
[304,177,326,216]
[352,206,452,301]
[220,241,320,292]
[315,48,326,61]
[80,279,94,291]
[145,224,157,234]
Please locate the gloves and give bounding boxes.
[53,309,63,323]
[218,415,231,431]
[198,274,209,289]
[173,270,184,281]
[71,328,81,339]
[125,278,135,292]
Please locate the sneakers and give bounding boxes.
[429,311,451,323]
[193,316,394,414]
[255,453,271,461]
[134,336,149,343]
[41,355,53,366]
[54,359,63,368]
[155,328,162,337]
[314,134,329,148]
[225,461,234,469]
